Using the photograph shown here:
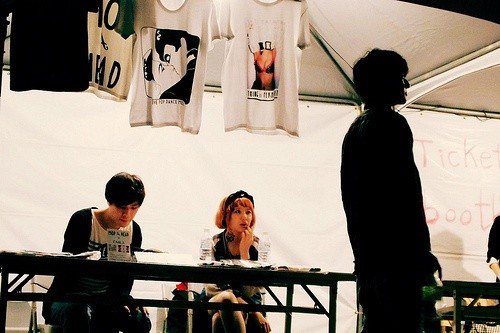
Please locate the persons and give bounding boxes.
[42,171,152,333]
[199,189,271,333]
[486,215,500,283]
[340,47,442,333]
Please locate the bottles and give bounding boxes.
[200,228,214,263]
[258,231,272,265]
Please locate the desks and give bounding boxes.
[0,253,357,333]
[438,280,500,333]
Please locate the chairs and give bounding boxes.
[21,275,63,333]
[187,282,266,333]
